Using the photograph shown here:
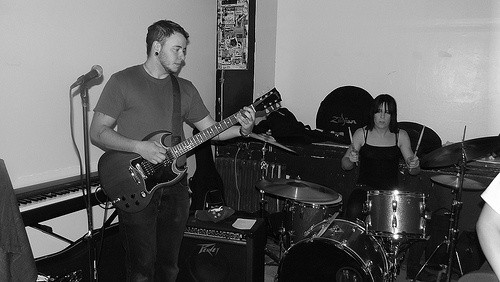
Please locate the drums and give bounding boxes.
[274,214,389,282]
[362,190,431,238]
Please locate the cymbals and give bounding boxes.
[431,172,487,191]
[255,174,344,206]
[248,129,296,153]
[416,135,500,170]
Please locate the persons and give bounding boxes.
[91,20,256,282]
[458,172,500,282]
[340,94,421,223]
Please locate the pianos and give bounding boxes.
[10,171,114,228]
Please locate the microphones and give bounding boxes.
[70,65,103,89]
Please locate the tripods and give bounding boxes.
[411,155,464,282]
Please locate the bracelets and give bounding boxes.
[239,127,253,138]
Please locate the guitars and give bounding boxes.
[97,87,282,215]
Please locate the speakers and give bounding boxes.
[175,215,267,282]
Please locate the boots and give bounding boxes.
[406,240,436,282]
